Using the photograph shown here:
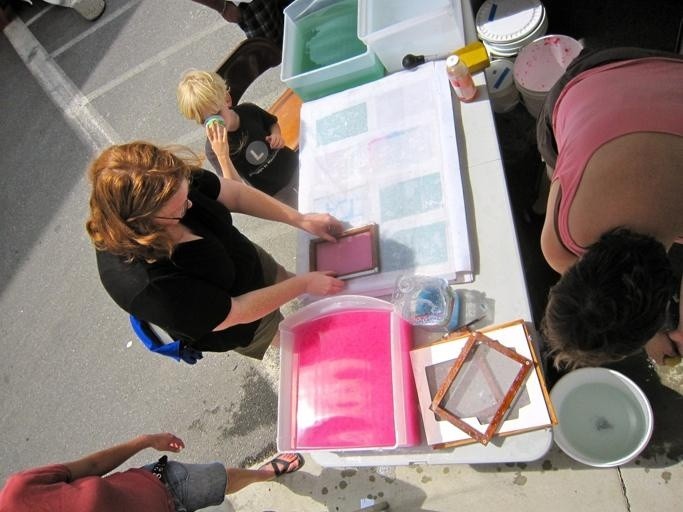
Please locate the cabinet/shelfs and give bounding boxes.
[391,273,461,334]
[445,54,479,103]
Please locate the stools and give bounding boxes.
[152,455,167,483]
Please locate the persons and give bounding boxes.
[86,143,346,361]
[175,68,299,210]
[0,431,305,512]
[537,43,683,374]
[198,2,284,43]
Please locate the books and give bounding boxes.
[276,294,420,452]
[280,0,386,102]
[357,0,466,73]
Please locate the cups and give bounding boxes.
[149,175,193,220]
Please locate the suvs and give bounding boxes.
[551,367,654,468]
[485,59,518,113]
[477,0,548,58]
[513,35,584,120]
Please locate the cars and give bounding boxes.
[203,115,227,136]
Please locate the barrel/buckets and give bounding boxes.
[258,453,302,478]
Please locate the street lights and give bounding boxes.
[130,314,202,364]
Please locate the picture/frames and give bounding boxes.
[295,0,553,468]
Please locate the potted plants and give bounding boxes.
[428,330,535,447]
[308,224,380,280]
[409,319,556,450]
